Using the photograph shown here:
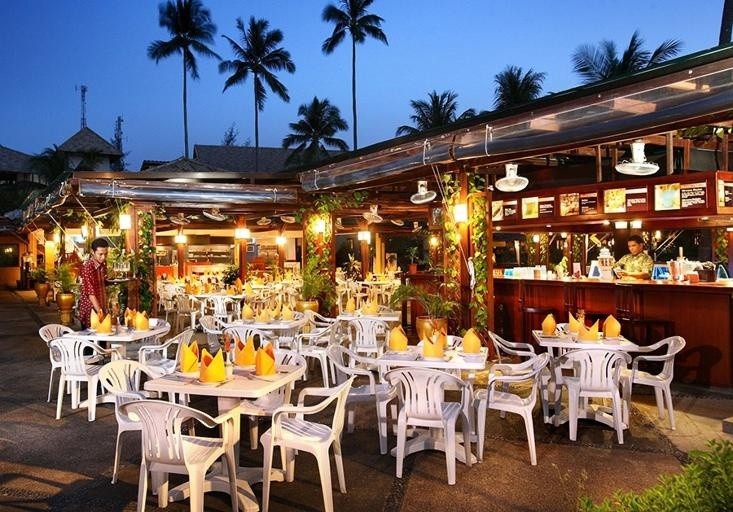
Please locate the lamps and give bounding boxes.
[186,209,206,221]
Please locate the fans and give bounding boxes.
[391,219,404,226]
[256,216,272,227]
[411,222,423,233]
[614,138,659,176]
[410,180,436,204]
[332,217,344,231]
[202,208,227,222]
[495,164,528,193]
[362,204,384,224]
[169,213,191,227]
[280,216,297,224]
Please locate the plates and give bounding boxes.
[242,316,295,326]
[84,326,153,337]
[385,341,483,362]
[540,328,620,343]
[176,361,292,385]
[342,310,381,317]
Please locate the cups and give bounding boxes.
[705,270,716,282]
[698,270,706,281]
[533,270,540,278]
[665,258,700,283]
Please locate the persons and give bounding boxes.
[613,234,654,280]
[78,238,115,355]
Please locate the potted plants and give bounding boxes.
[27,265,50,308]
[392,267,461,341]
[293,256,332,312]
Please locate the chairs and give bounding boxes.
[553,347,633,445]
[325,342,397,455]
[346,317,390,382]
[39,324,104,403]
[118,399,241,511]
[473,353,552,467]
[304,309,342,334]
[335,264,409,313]
[47,336,124,422]
[295,318,343,388]
[156,269,305,334]
[259,374,357,510]
[137,327,196,403]
[385,366,472,485]
[555,322,594,335]
[610,335,686,430]
[97,358,197,485]
[488,331,549,424]
[201,313,280,366]
[111,317,172,362]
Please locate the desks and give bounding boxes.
[374,345,490,457]
[143,362,286,512]
[62,324,168,409]
[229,319,310,330]
[337,310,404,323]
[532,329,640,432]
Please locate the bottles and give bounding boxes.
[111,310,117,334]
